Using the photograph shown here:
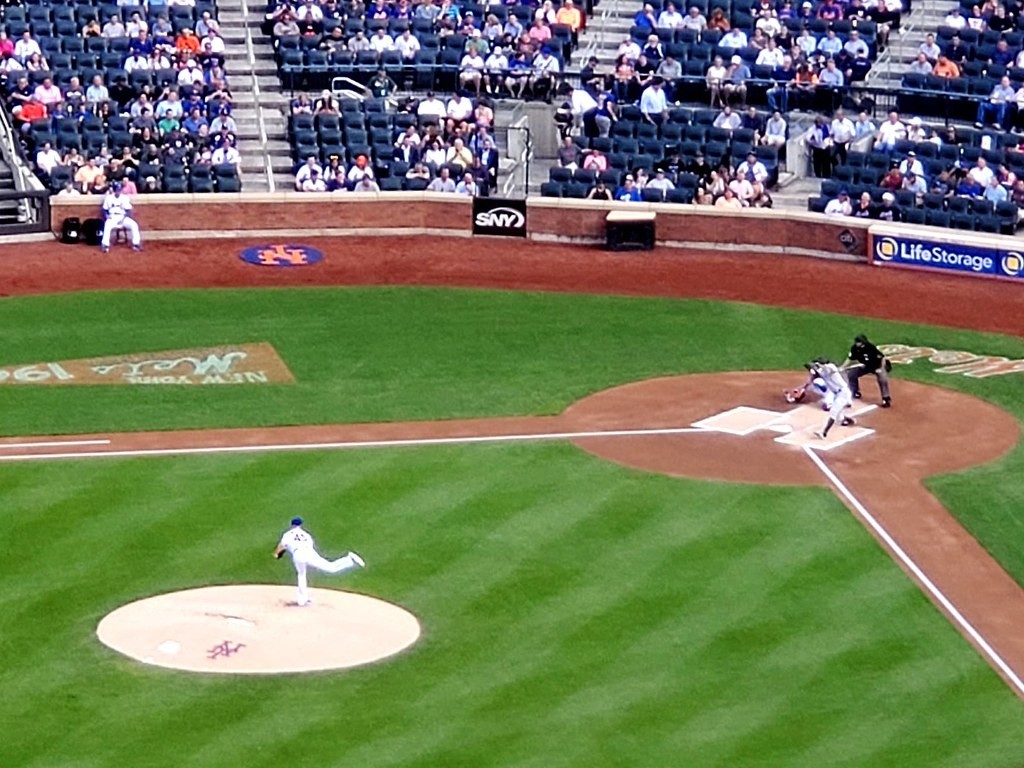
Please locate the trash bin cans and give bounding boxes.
[84,217,105,245]
[61,217,80,244]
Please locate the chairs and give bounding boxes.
[0,0,1024,244]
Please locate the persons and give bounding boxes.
[0,0,1024,228]
[100,182,140,252]
[839,334,892,408]
[272,517,365,606]
[799,357,857,440]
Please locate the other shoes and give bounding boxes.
[133,245,140,251]
[349,552,366,568]
[305,600,311,604]
[854,393,861,398]
[882,399,890,407]
[844,417,857,425]
[814,431,826,440]
[102,246,109,252]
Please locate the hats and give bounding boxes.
[113,181,122,192]
[292,518,303,526]
[812,357,828,363]
[857,335,867,343]
